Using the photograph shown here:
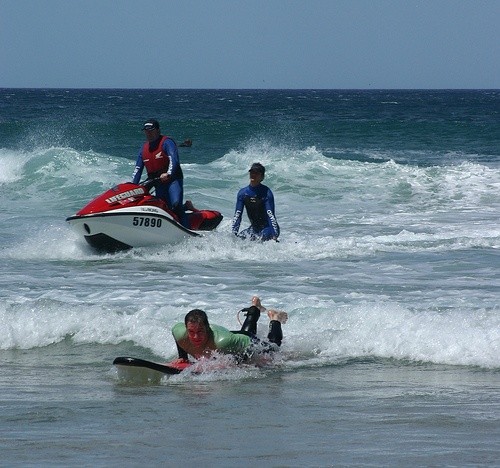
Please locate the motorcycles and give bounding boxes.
[66,175,224,251]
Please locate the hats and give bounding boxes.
[249,162,265,172]
[142,119,160,130]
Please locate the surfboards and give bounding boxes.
[113,357,271,375]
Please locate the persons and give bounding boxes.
[232,162,280,243]
[172,297,288,368]
[131,119,201,215]
